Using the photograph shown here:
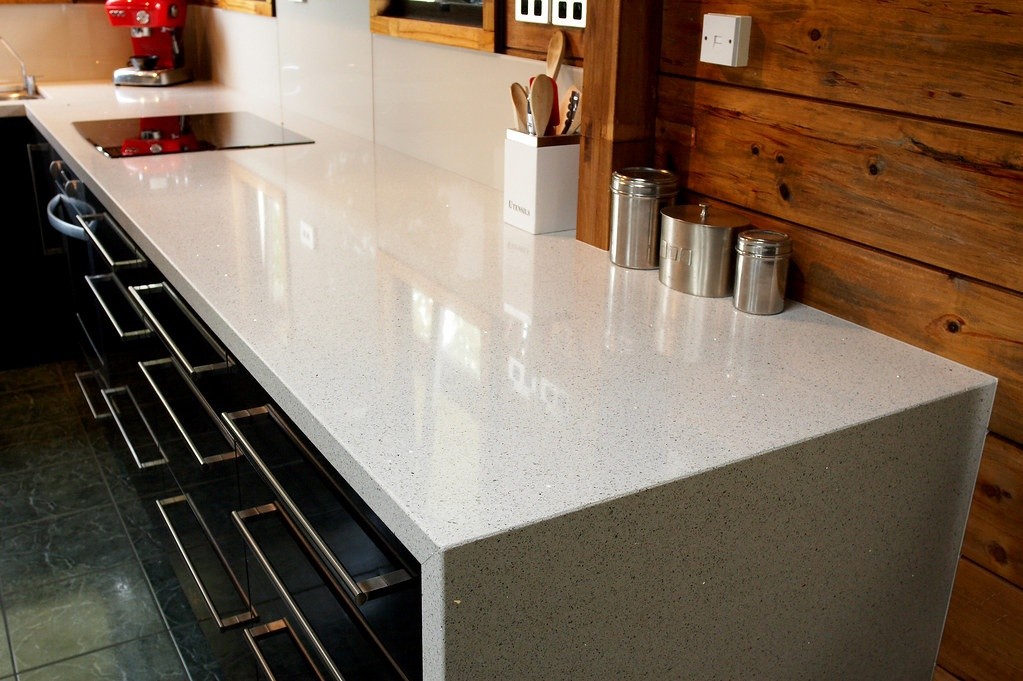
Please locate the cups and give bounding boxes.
[659,202,751,295]
[127,56,160,71]
[503,128,581,235]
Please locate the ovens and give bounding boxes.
[47,160,98,326]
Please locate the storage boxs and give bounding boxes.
[503,128,580,235]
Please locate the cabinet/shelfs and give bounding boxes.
[369,0,588,68]
[26,141,424,681]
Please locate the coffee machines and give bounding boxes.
[104,0,189,86]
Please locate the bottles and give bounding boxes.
[609,167,684,270]
[732,231,792,314]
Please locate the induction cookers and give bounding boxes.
[73,110,315,158]
